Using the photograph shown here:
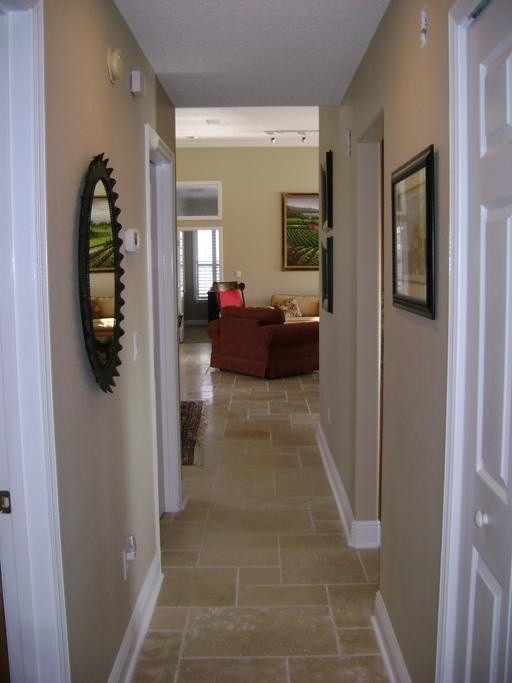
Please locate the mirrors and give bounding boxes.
[78,152,125,393]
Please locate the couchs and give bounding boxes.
[91,297,115,339]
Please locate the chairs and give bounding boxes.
[207,281,320,379]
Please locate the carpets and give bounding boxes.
[180,400,208,465]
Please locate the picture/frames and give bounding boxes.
[391,143,435,321]
[89,196,115,273]
[176,181,223,220]
[322,150,333,314]
[282,193,320,271]
